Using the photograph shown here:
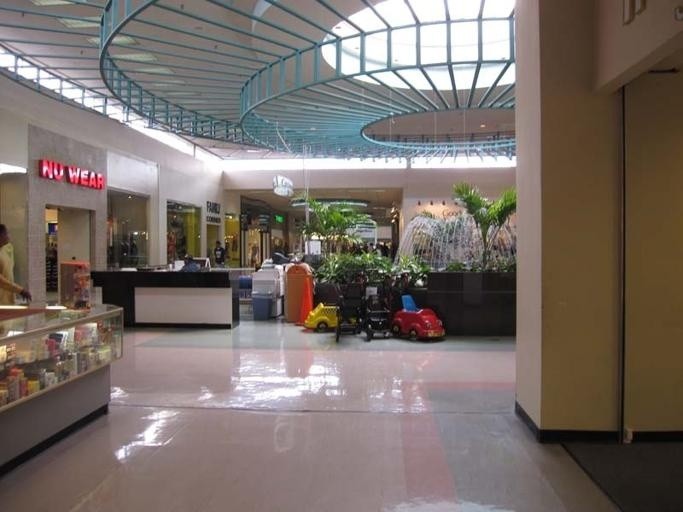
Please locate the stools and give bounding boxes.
[239,275,253,299]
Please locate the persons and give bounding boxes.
[121,232,139,267]
[349,240,390,258]
[0,224,33,302]
[178,253,200,272]
[213,240,225,268]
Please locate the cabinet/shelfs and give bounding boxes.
[0,300,124,478]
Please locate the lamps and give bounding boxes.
[273,174,294,198]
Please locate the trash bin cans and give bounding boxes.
[251,291,273,320]
[284,262,313,323]
